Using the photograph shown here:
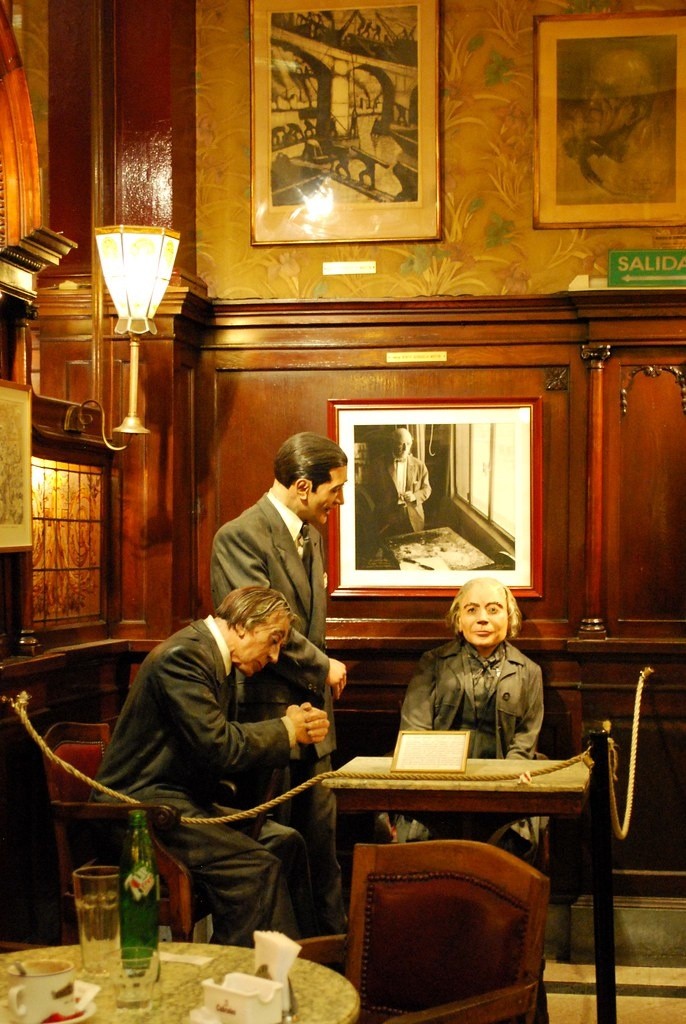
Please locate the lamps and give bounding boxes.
[62,225,180,453]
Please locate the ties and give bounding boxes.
[300,522,312,583]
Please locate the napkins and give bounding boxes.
[253,930,304,1012]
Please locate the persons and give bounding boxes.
[86,586,330,949]
[363,428,432,540]
[211,432,348,941]
[373,578,549,865]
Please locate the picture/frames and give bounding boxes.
[249,0,442,247]
[325,398,545,600]
[533,8,686,230]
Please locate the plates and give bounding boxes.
[50,1000,98,1024]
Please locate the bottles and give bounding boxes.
[118,809,161,983]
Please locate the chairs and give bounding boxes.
[294,838,549,1023]
[30,721,211,948]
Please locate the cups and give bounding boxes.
[72,865,120,985]
[6,959,76,1023]
[103,946,159,1016]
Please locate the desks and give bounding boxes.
[320,756,592,964]
[1,938,361,1023]
